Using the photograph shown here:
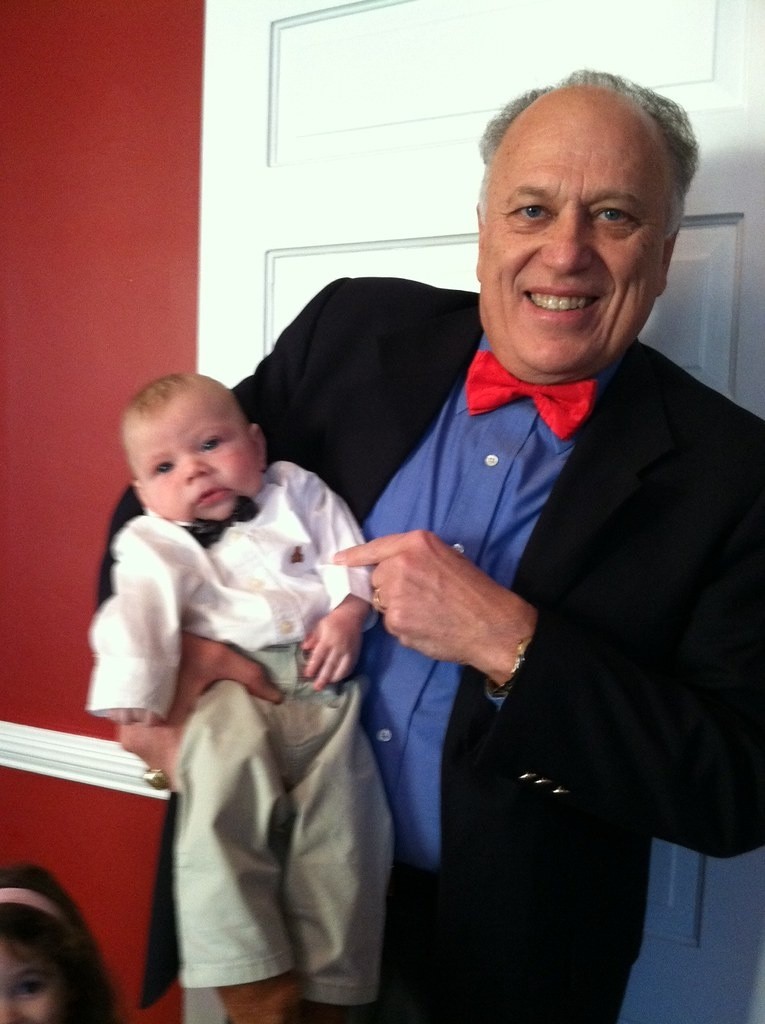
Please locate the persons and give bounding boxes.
[0,862,118,1024]
[92,70,765,1024]
[83,370,398,1024]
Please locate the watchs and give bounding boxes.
[483,634,535,701]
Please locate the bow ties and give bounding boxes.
[187,495,259,546]
[465,348,597,440]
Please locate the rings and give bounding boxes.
[372,587,386,615]
[140,768,170,793]
